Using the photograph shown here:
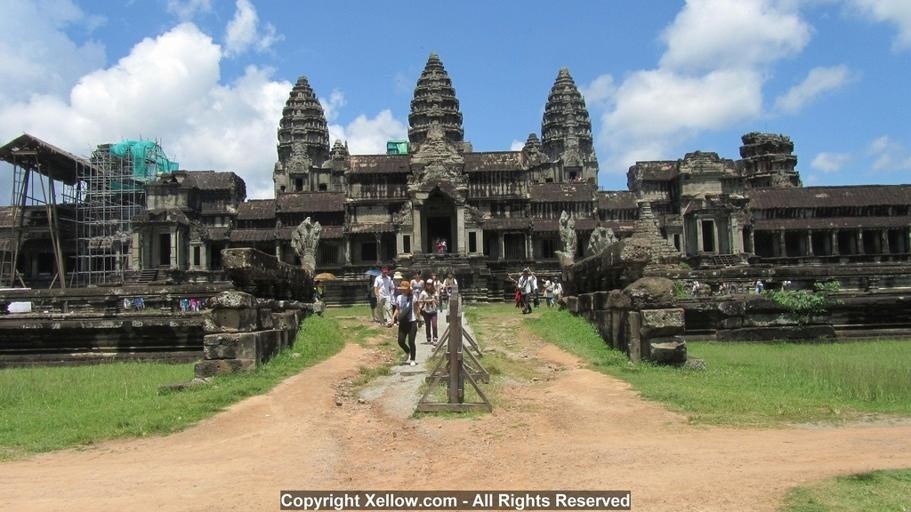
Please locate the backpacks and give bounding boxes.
[422,292,437,314]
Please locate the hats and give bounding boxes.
[399,281,409,290]
[382,269,389,273]
[392,271,403,279]
[426,278,433,284]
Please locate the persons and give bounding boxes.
[434,238,449,254]
[366,267,459,327]
[122,296,146,312]
[418,278,439,346]
[691,280,792,298]
[314,280,323,301]
[506,266,564,314]
[178,295,207,312]
[390,281,422,366]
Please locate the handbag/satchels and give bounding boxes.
[441,289,447,300]
[516,291,521,302]
[398,306,410,322]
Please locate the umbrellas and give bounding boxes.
[314,273,338,281]
